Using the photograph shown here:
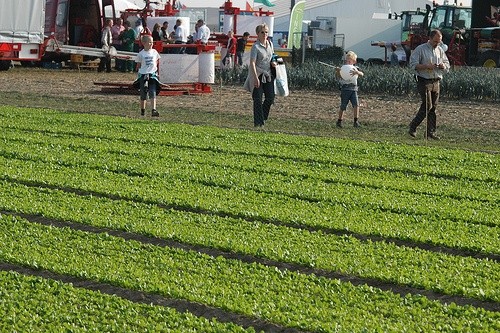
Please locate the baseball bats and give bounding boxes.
[318,62,341,70]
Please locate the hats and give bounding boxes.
[154,23,160,30]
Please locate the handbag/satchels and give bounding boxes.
[274,64,290,97]
[109,46,118,59]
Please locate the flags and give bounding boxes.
[246,1,253,11]
[254,0,277,7]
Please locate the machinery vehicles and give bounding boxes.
[387,0,500,69]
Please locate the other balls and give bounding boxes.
[340,65,358,80]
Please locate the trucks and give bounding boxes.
[0,30,61,72]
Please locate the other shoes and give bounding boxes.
[140,114,146,118]
[336,120,344,129]
[353,121,362,127]
[409,124,416,137]
[424,130,440,141]
[151,111,160,117]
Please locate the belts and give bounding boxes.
[417,76,440,81]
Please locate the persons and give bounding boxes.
[134,18,186,53]
[135,35,161,117]
[193,19,211,44]
[110,17,126,71]
[336,50,365,128]
[280,34,288,47]
[408,29,451,140]
[97,18,115,73]
[221,30,237,68]
[236,32,249,67]
[249,24,277,128]
[118,20,137,73]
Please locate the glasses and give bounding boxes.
[260,31,268,34]
[431,38,442,44]
